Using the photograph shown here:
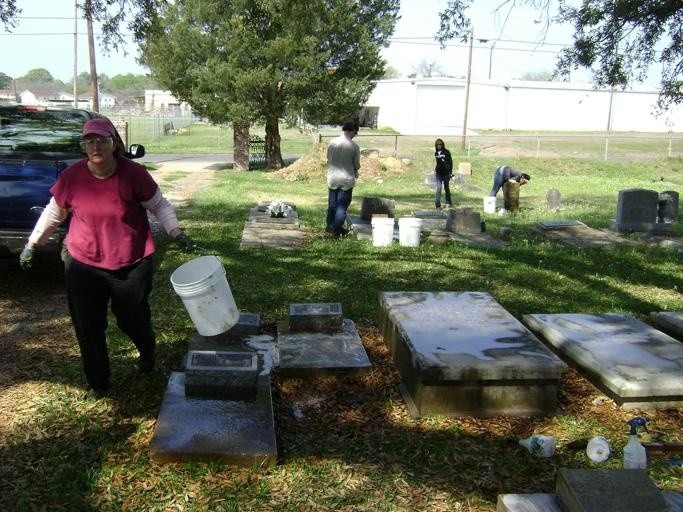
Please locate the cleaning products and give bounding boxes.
[624,416,648,471]
[512,435,557,457]
[585,436,610,462]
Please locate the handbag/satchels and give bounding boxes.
[56,234,70,262]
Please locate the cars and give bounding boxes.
[1,103,146,255]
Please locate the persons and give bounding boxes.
[488,165,530,209]
[325,123,360,234]
[434,138,452,208]
[18,118,193,401]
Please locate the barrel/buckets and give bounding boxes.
[397,218,423,247]
[484,196,497,213]
[372,216,395,248]
[170,244,239,338]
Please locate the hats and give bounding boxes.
[83,118,115,136]
[343,120,359,131]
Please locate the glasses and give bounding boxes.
[85,138,111,144]
[436,143,442,146]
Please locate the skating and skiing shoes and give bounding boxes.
[437,204,451,210]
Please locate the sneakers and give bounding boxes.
[140,349,156,372]
[325,226,350,235]
[86,375,110,400]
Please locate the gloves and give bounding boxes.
[175,233,197,254]
[20,244,40,272]
